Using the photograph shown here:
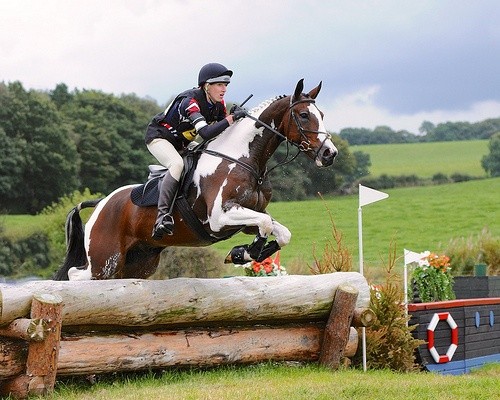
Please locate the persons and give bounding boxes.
[142,62,250,241]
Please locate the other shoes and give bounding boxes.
[151,214,174,241]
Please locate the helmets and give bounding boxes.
[198,62,233,87]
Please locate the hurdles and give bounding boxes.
[0,271,378,399]
[400,297,500,376]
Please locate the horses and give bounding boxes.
[53,78,338,282]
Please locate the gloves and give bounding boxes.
[232,110,246,122]
[230,104,245,114]
